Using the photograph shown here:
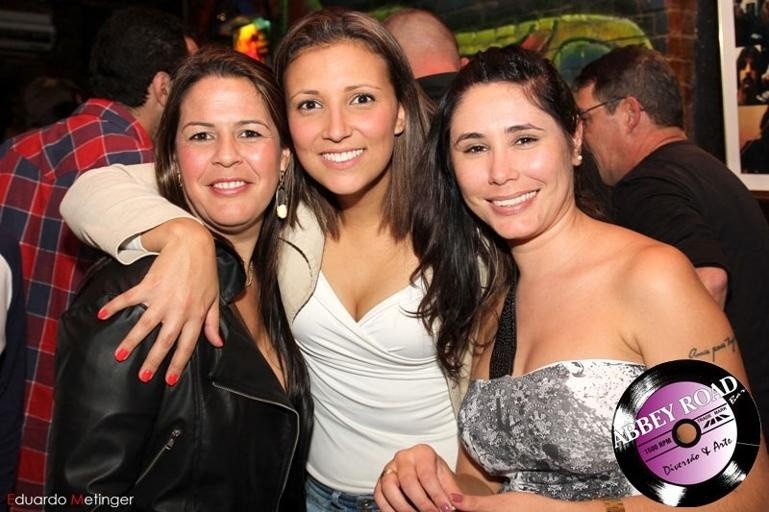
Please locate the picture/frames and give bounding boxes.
[716,1,769,192]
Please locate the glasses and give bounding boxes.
[577,96,646,130]
